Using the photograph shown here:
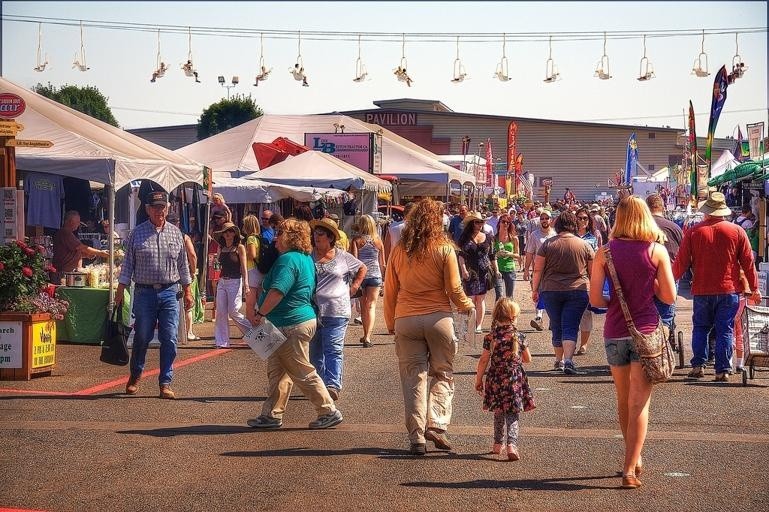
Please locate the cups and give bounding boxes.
[213,258,220,271]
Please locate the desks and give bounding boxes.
[55,286,130,345]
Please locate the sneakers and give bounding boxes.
[475,316,730,382]
[426,429,451,450]
[125,375,141,394]
[622,455,643,488]
[160,385,175,398]
[246,317,374,428]
[410,444,426,455]
[493,443,505,454]
[507,443,521,461]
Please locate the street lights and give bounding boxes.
[218,75,239,100]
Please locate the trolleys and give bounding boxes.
[667,328,685,369]
[741,293,769,385]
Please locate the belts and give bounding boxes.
[222,276,241,280]
[135,283,174,289]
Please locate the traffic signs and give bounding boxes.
[5,140,54,148]
[0,122,24,130]
[1,130,18,136]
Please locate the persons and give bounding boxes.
[150,63,164,83]
[728,62,745,85]
[393,65,413,87]
[588,195,678,490]
[112,190,198,399]
[186,60,201,84]
[474,297,536,461]
[50,210,110,285]
[253,66,265,86]
[441,194,616,376]
[246,218,343,429]
[383,196,475,454]
[647,190,761,382]
[210,192,416,349]
[294,64,310,88]
[101,219,121,245]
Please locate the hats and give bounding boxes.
[309,217,341,240]
[463,212,484,224]
[329,214,341,222]
[589,203,600,211]
[212,223,244,246]
[144,192,167,206]
[214,211,225,218]
[540,211,551,217]
[698,191,731,217]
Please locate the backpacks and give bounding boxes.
[246,235,273,274]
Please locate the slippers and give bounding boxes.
[187,335,200,341]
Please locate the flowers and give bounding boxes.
[0,238,69,326]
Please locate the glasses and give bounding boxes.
[578,216,589,221]
[540,217,550,220]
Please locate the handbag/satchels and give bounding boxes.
[100,320,133,366]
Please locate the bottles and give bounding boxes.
[65,268,87,287]
[499,242,504,259]
[78,233,101,248]
